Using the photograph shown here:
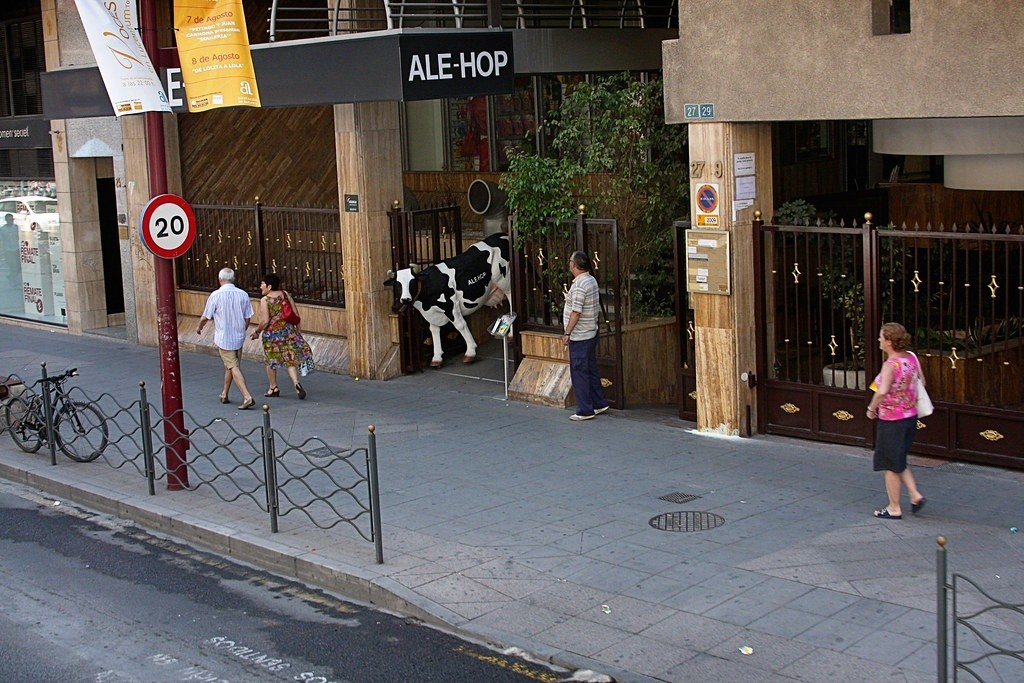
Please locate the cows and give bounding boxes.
[383,232,513,369]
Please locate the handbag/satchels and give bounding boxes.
[298,357,315,376]
[906,351,935,418]
[281,291,298,325]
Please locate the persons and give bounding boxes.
[0,214,20,287]
[562,252,609,421]
[867,323,928,519]
[250,274,315,400]
[197,268,255,410]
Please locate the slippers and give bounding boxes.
[911,497,927,513]
[874,506,902,519]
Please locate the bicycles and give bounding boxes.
[5,369,108,464]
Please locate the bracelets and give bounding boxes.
[868,406,876,412]
[198,328,202,331]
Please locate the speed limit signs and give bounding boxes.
[140,194,196,259]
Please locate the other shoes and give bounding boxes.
[238,397,256,409]
[594,406,609,414]
[219,393,228,403]
[569,413,596,421]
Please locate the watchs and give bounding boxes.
[256,330,260,335]
[564,332,571,336]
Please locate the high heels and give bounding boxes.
[295,384,307,401]
[263,387,280,397]
